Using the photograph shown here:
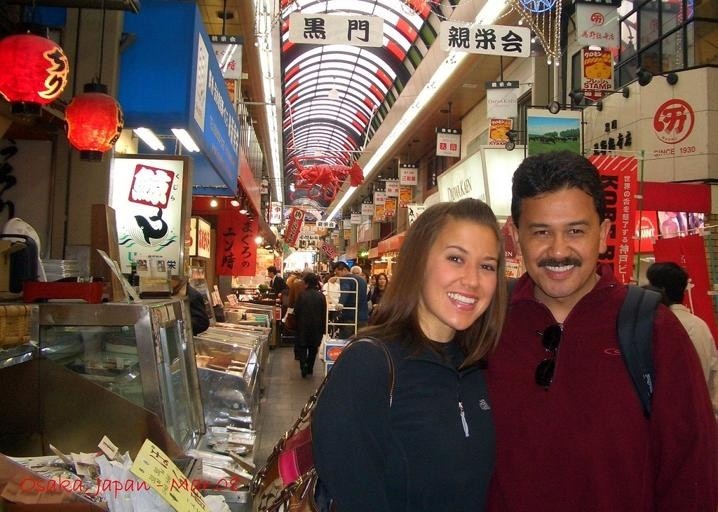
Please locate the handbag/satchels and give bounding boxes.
[281,307,297,331]
[247,425,322,512]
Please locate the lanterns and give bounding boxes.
[63,83,124,163]
[0,25,70,117]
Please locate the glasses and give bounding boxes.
[534,323,563,392]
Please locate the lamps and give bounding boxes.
[548,62,677,114]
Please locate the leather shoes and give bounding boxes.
[294,355,313,378]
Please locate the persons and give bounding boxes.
[186,280,210,338]
[647,261,718,420]
[312,198,508,512]
[482,151,718,511]
[267,261,392,377]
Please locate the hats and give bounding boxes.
[267,265,276,272]
